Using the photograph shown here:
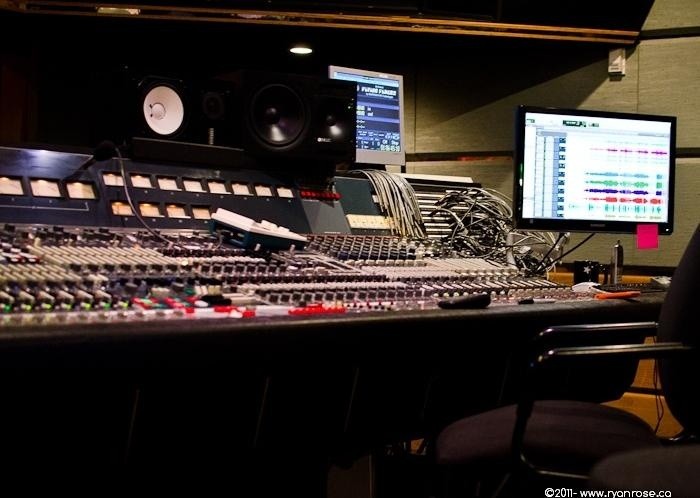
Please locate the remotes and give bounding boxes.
[439,293,491,309]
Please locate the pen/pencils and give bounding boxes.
[518,298,556,305]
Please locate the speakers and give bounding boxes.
[130,55,357,170]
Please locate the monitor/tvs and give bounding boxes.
[328,66,406,173]
[513,105,676,235]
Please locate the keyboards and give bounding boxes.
[593,281,665,293]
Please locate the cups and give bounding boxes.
[572,260,608,287]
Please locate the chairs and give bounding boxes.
[436,224,700,497]
